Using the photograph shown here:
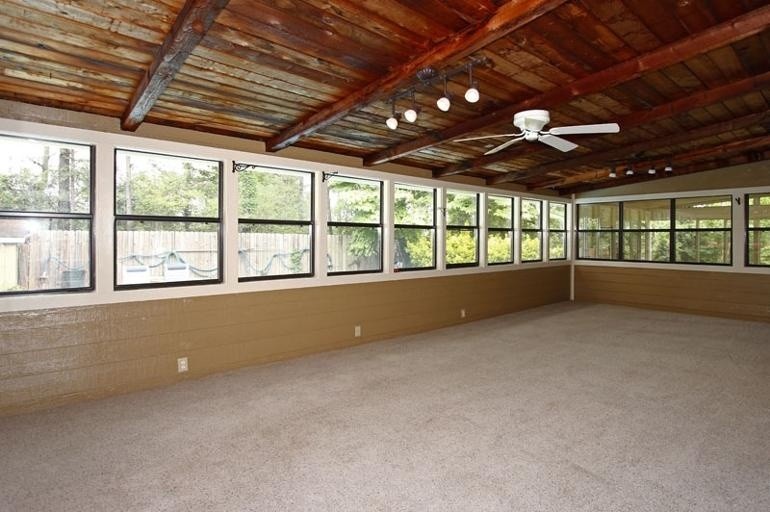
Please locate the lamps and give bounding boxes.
[607,152,673,180]
[380,56,481,130]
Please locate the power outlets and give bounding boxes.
[354,325,362,337]
[176,355,189,373]
[459,308,466,317]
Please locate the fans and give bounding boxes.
[452,109,621,157]
